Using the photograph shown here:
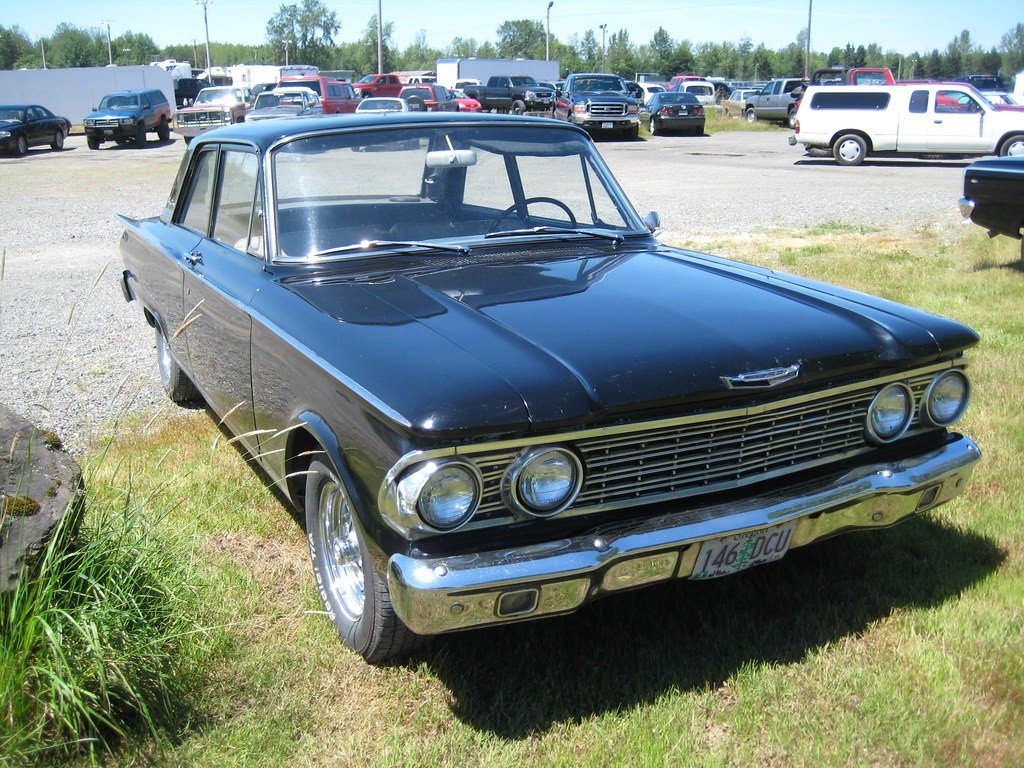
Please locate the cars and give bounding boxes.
[623,70,768,118]
[956,92,1024,112]
[958,156,1024,268]
[0,77,482,159]
[940,75,1008,101]
[636,91,705,136]
[553,74,639,141]
[113,111,983,665]
[897,80,959,112]
[536,79,564,91]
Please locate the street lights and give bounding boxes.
[34,34,48,69]
[282,40,292,66]
[100,19,116,66]
[195,0,214,86]
[600,24,607,73]
[546,2,554,61]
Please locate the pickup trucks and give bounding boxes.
[743,79,812,129]
[350,74,407,100]
[789,82,1024,167]
[463,75,555,115]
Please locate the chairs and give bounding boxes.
[232,223,387,251]
[9,112,20,120]
[392,217,527,241]
